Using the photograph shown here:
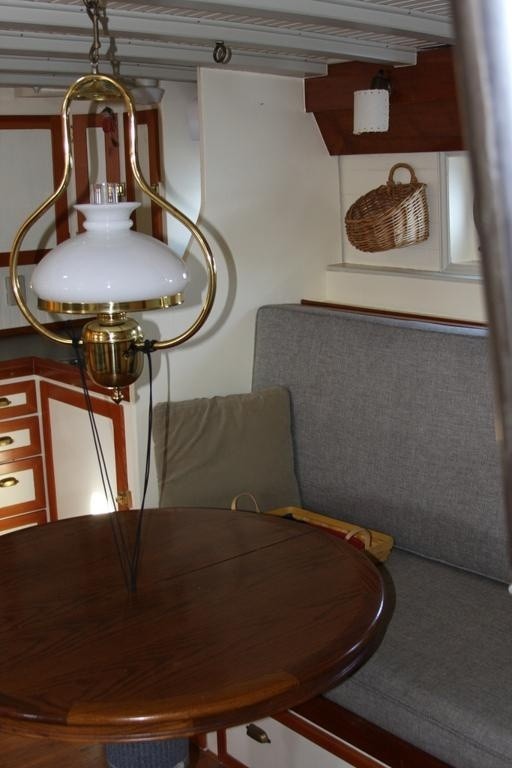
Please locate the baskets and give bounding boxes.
[230,492,394,566]
[345,162,429,253]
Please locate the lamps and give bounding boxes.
[8,0,221,610]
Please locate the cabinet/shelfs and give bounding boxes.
[0,375,49,536]
[37,375,137,524]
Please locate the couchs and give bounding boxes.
[183,298,512,768]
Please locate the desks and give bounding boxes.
[0,507,395,768]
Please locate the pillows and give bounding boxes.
[151,385,304,524]
